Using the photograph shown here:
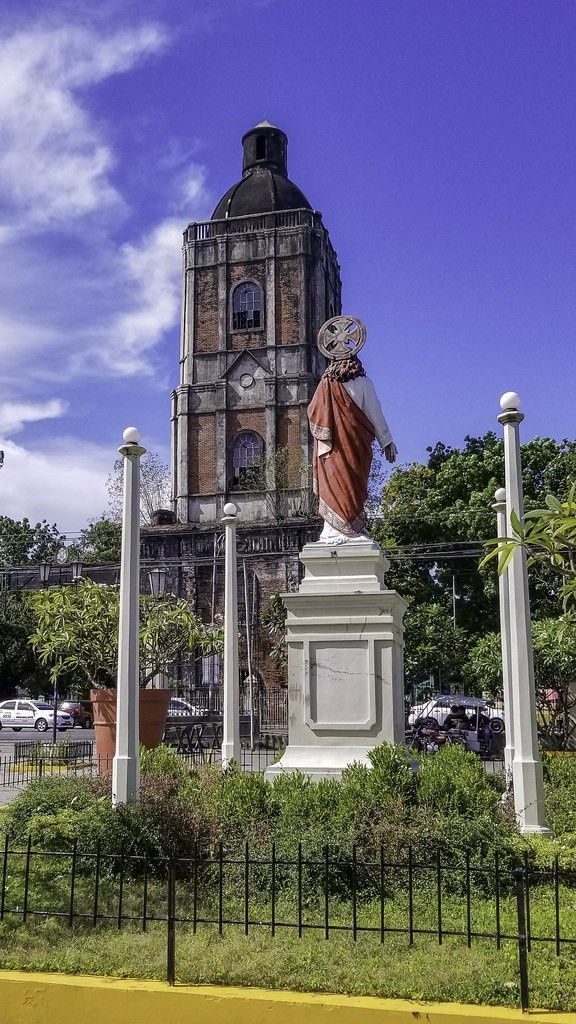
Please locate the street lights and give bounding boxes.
[491,487,516,802]
[112,426,148,805]
[495,389,556,841]
[220,501,242,770]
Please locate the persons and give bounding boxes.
[442,705,470,731]
[307,347,398,538]
[458,705,468,719]
[470,707,488,726]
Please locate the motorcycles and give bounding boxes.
[406,717,468,753]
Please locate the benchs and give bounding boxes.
[435,722,469,743]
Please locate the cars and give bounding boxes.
[58,700,94,729]
[166,698,223,716]
[408,697,505,734]
[0,700,74,732]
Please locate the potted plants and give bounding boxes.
[27,577,225,775]
